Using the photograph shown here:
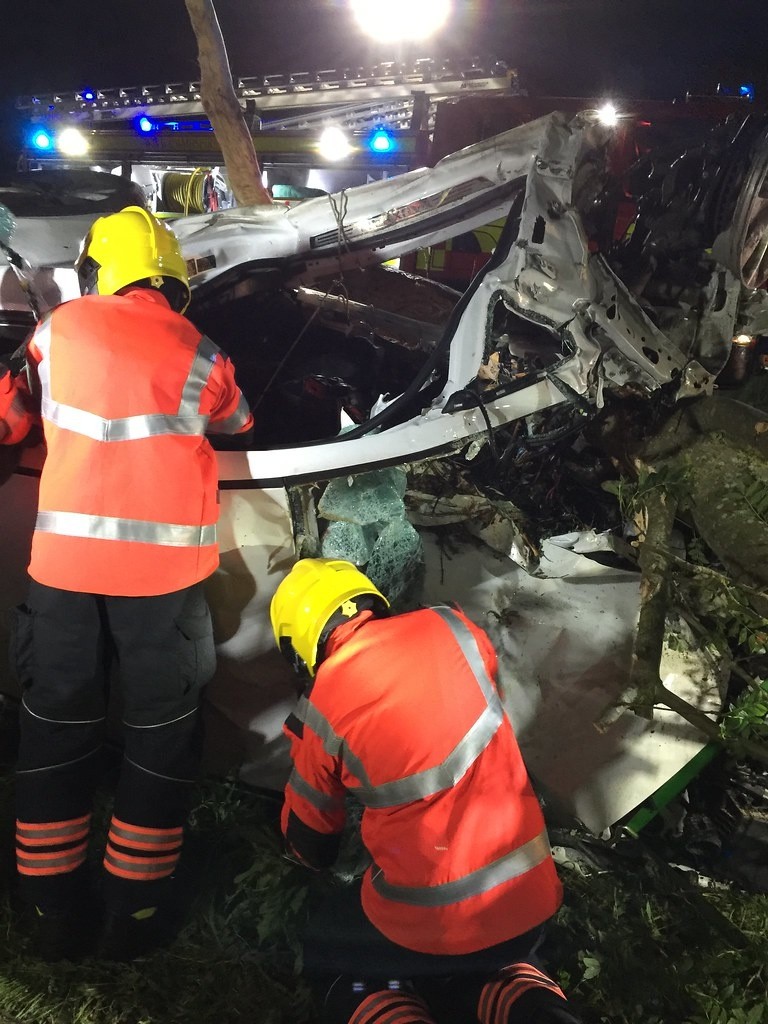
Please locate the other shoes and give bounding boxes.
[13,875,193,961]
[507,989,582,1024]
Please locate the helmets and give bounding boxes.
[73,206,192,315]
[269,557,391,680]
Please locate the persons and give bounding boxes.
[2,207,257,950]
[268,557,587,1023]
